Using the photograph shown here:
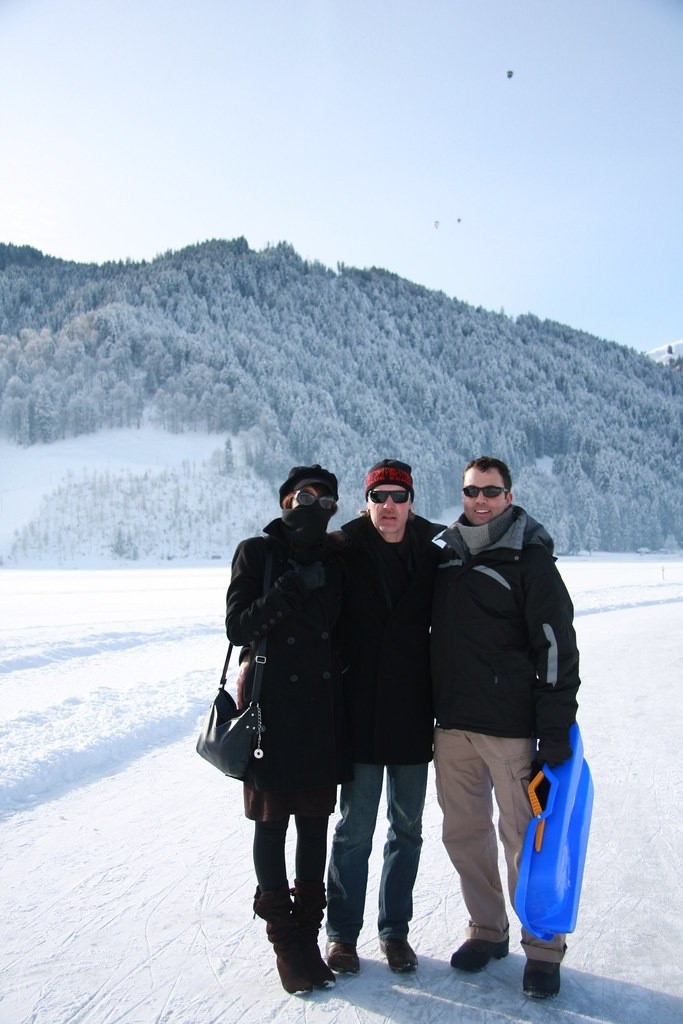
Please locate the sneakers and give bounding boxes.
[522,957,561,999]
[327,940,359,972]
[451,937,509,971]
[380,937,418,973]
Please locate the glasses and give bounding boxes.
[462,485,509,498]
[296,491,334,510]
[369,490,409,504]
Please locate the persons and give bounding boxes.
[225,455,582,1001]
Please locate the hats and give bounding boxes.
[279,464,339,507]
[364,459,415,501]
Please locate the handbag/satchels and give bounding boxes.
[197,689,272,781]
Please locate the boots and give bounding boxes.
[254,880,312,995]
[291,878,336,988]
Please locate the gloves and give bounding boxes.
[289,562,324,591]
[539,727,573,770]
